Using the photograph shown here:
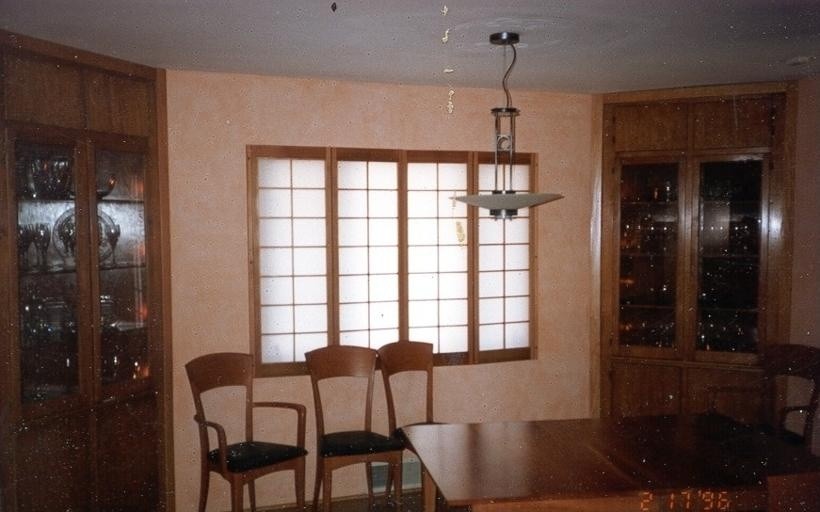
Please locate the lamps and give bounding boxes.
[447,30,564,220]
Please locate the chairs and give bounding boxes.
[184,352,308,512]
[377,342,451,512]
[305,346,406,512]
[715,345,820,453]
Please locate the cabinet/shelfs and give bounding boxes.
[0,32,177,512]
[594,79,802,416]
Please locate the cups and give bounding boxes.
[105,224,120,266]
[32,223,52,270]
[16,224,32,272]
[57,223,73,263]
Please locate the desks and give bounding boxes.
[392,416,820,512]
